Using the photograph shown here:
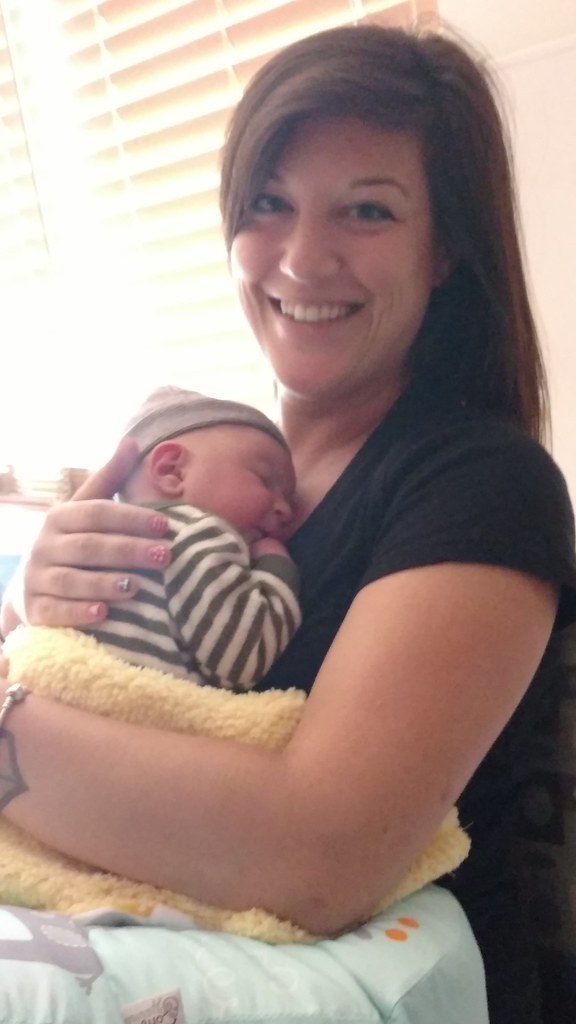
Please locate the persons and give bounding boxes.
[0,28,576,1024]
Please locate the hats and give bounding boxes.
[112,384,290,462]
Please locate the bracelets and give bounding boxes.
[0,683,33,728]
